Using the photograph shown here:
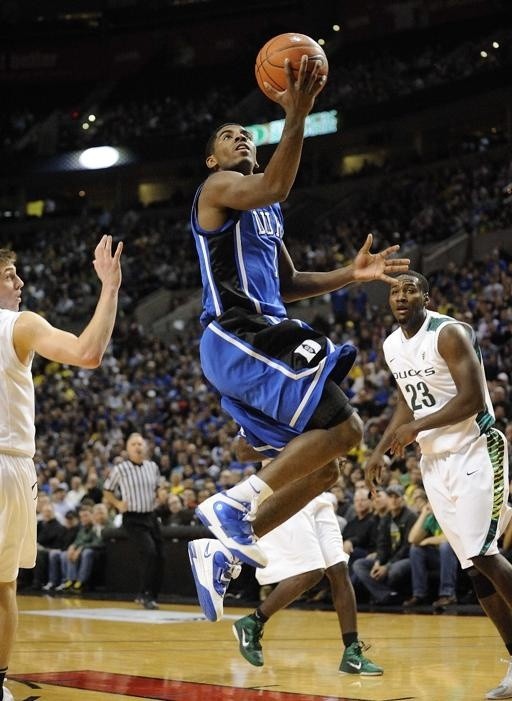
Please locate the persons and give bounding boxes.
[183,51,409,626]
[363,268,511,701]
[1,231,127,701]
[230,421,388,679]
[1,48,512,609]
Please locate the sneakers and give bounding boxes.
[403,596,430,607]
[433,595,458,607]
[232,614,263,665]
[339,642,383,676]
[195,490,268,568]
[144,599,160,610]
[188,538,242,623]
[32,579,83,592]
[487,656,512,700]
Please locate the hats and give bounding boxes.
[386,484,406,497]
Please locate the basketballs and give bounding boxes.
[255,32,328,101]
